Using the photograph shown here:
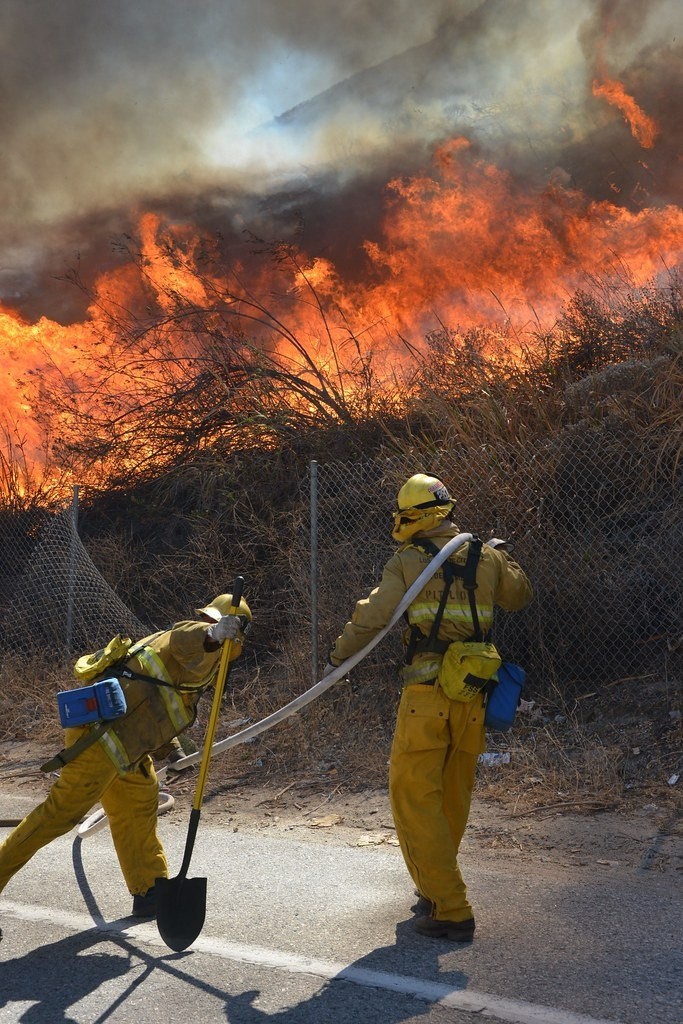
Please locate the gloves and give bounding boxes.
[485,537,515,553]
[207,615,241,641]
[324,665,350,686]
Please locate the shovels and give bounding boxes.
[150,577,243,954]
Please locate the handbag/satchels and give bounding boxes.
[439,641,502,702]
[56,677,126,728]
[483,661,526,731]
[73,634,132,681]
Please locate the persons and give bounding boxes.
[0,593,253,938]
[323,474,533,941]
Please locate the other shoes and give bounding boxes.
[132,888,158,917]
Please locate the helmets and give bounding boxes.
[397,473,457,510]
[195,594,253,642]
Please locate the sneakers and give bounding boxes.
[414,888,432,911]
[413,914,475,941]
